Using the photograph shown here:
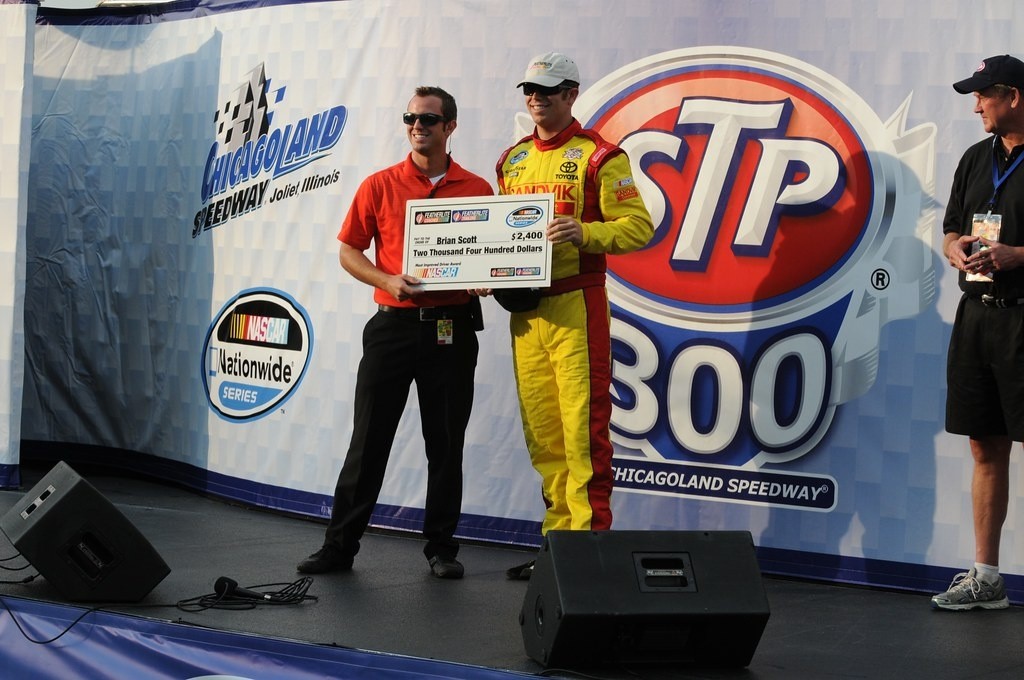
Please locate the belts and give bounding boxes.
[966,292,1024,308]
[378,303,469,322]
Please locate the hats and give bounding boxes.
[953,54,1024,94]
[516,51,580,88]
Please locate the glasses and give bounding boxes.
[523,83,576,97]
[403,113,448,127]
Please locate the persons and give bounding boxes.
[929,55,1024,611]
[294,87,495,579]
[495,52,654,579]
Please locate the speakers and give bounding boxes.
[519,531,771,675]
[0,460,172,608]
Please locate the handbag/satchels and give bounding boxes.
[470,295,484,331]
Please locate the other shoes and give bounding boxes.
[507,559,535,582]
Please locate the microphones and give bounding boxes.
[213,576,279,603]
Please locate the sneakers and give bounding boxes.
[297,547,354,575]
[930,566,1009,611]
[431,555,464,580]
